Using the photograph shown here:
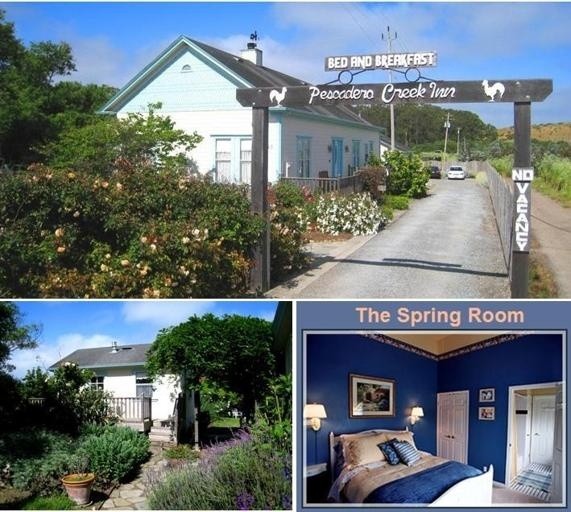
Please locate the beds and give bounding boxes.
[329,427,496,505]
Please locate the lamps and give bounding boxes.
[305,402,329,432]
[407,406,425,429]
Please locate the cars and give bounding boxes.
[429,167,440,178]
[448,166,466,180]
[227,408,242,418]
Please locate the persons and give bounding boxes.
[363,387,390,402]
[480,409,492,418]
[481,390,492,400]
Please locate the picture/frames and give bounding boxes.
[349,372,397,419]
[478,388,497,403]
[477,405,497,421]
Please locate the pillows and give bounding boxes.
[377,438,402,465]
[393,440,422,467]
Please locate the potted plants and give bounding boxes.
[62,454,97,506]
